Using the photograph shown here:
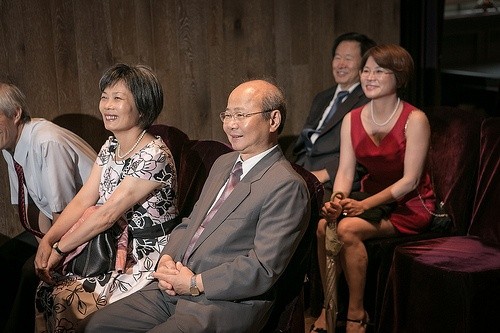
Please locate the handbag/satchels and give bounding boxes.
[67,220,124,279]
[421,201,450,238]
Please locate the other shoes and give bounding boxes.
[311,306,328,333]
[345,311,370,333]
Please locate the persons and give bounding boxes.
[35,63,180,333]
[76,79,312,333]
[287,31,378,190]
[0,83,100,333]
[311,43,435,333]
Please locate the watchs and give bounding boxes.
[53,242,66,256]
[190,275,200,296]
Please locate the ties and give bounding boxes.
[15,162,45,240]
[301,90,348,157]
[182,160,243,266]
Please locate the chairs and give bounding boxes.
[148,105,500,333]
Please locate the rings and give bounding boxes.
[343,213,347,215]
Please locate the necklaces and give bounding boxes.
[370,98,400,126]
[117,130,145,159]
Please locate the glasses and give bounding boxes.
[219,107,275,123]
